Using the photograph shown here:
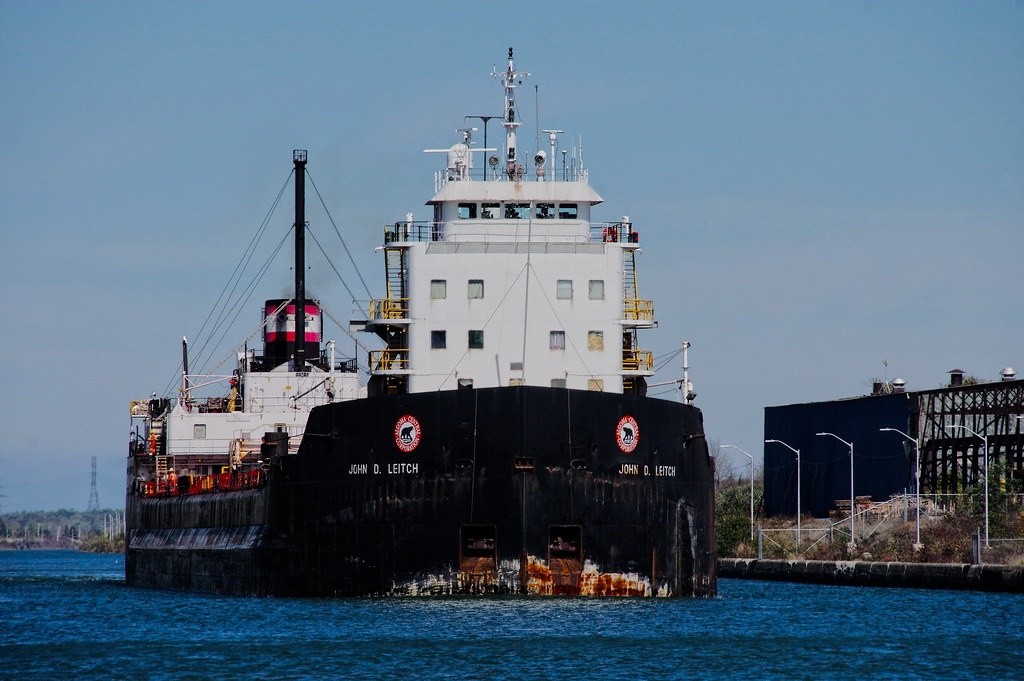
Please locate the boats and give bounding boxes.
[123,46,719,600]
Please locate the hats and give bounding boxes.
[167,468,174,471]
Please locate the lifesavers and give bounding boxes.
[602,227,617,242]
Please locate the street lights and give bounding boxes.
[765,439,802,547]
[720,444,754,541]
[816,433,854,543]
[880,428,920,545]
[946,425,990,548]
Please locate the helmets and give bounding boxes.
[149,431,154,433]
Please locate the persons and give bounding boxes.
[149,430,157,455]
[158,468,177,491]
